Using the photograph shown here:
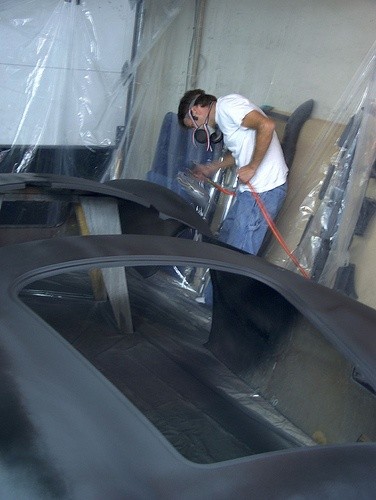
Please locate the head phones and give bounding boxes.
[194,99,223,144]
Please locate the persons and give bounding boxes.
[177,87,289,257]
